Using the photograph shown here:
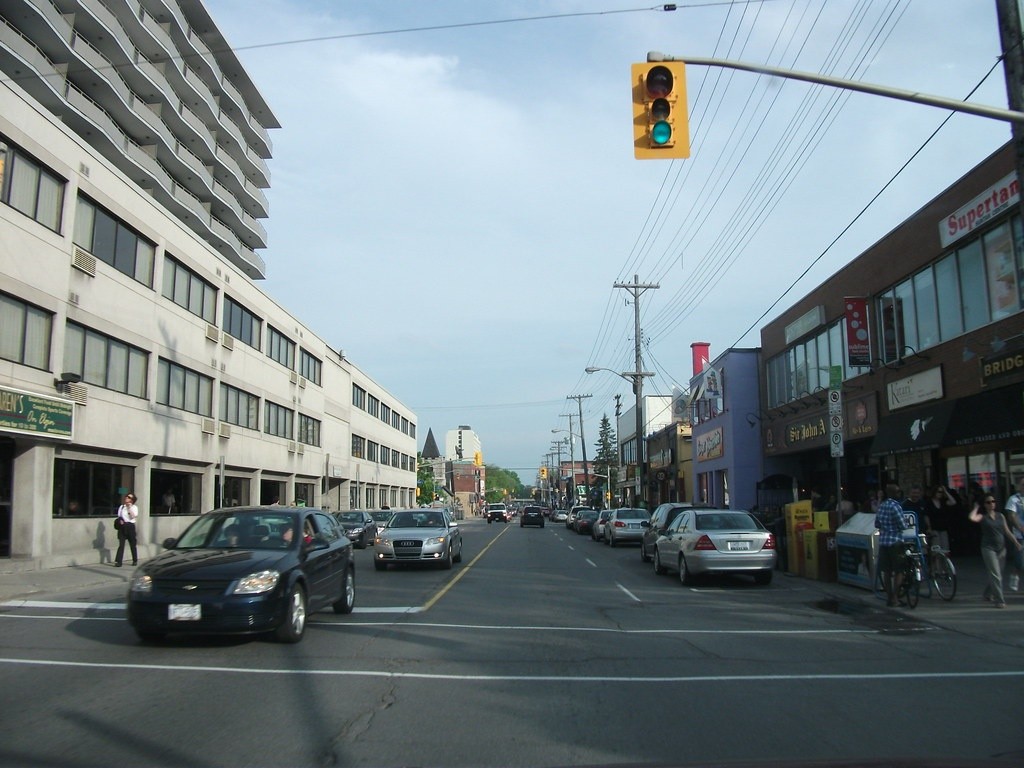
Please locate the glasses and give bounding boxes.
[984,499,995,505]
[936,490,943,493]
[126,495,132,499]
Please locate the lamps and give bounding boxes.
[746,412,764,428]
[700,413,713,424]
[712,406,728,417]
[814,386,827,406]
[899,346,928,367]
[787,398,809,413]
[775,401,799,418]
[991,327,1024,353]
[688,418,701,428]
[963,337,991,362]
[798,391,822,409]
[54,373,81,388]
[764,406,781,422]
[867,358,900,376]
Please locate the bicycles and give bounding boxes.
[880,523,957,610]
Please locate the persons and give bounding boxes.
[279,523,312,544]
[1005,476,1024,590]
[271,496,279,506]
[875,482,909,607]
[224,524,241,546]
[114,492,138,566]
[970,493,1022,608]
[810,486,883,513]
[901,479,957,569]
[382,502,390,510]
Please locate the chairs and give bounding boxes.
[337,515,344,523]
[400,515,415,527]
[700,517,713,528]
[233,526,251,547]
[435,514,441,524]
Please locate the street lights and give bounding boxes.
[551,428,591,510]
[585,366,644,508]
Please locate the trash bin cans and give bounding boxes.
[834,511,885,591]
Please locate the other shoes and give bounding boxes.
[1009,574,1019,590]
[115,563,122,567]
[132,561,137,566]
[942,570,951,580]
[984,593,995,600]
[996,602,1006,608]
[886,597,896,606]
[890,599,906,607]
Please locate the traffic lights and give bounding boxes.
[432,492,436,500]
[540,466,548,479]
[606,492,611,500]
[631,61,691,159]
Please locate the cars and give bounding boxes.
[374,507,463,570]
[592,510,615,542]
[572,510,600,535]
[126,505,356,646]
[541,506,559,522]
[330,509,378,549]
[553,509,570,523]
[603,507,652,548]
[481,504,523,522]
[358,507,405,536]
[653,508,778,587]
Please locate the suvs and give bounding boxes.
[486,502,509,523]
[640,501,720,563]
[564,505,593,530]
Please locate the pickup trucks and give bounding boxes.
[519,506,546,528]
